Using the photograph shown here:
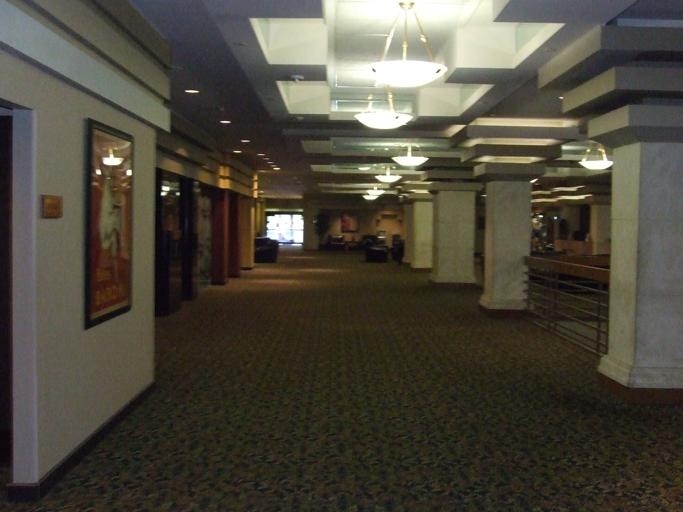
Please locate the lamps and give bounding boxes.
[578,145,612,171]
[363,1,450,88]
[374,166,400,183]
[351,93,414,131]
[391,143,428,166]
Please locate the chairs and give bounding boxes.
[326,234,404,264]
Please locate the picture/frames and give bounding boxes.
[83,117,136,331]
